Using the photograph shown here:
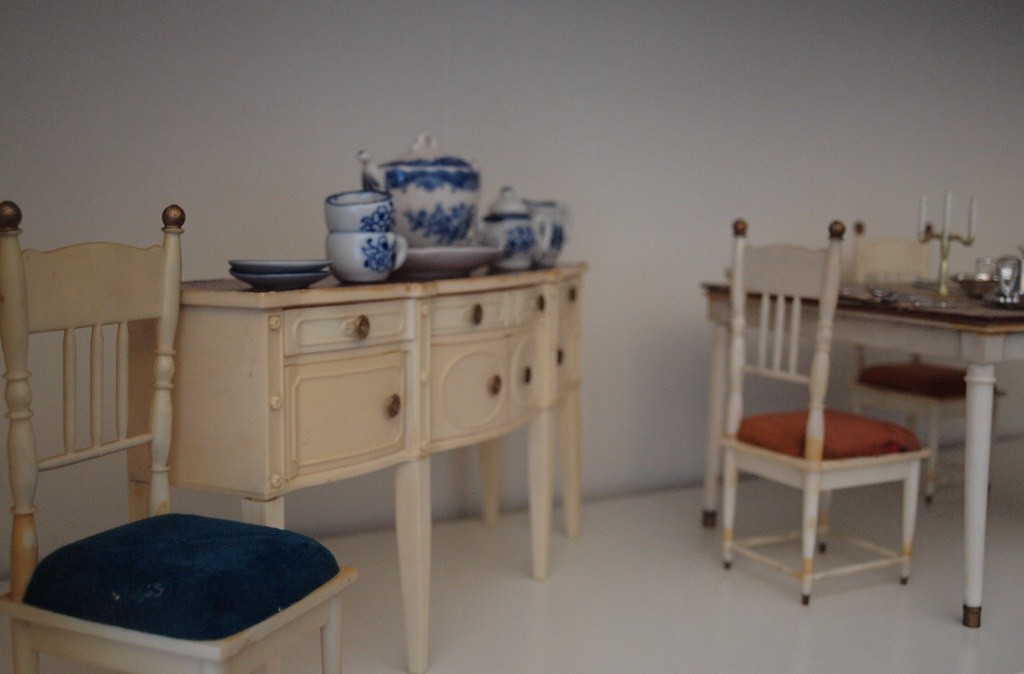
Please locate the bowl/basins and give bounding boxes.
[952,271,1001,299]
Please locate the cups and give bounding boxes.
[324,190,396,232]
[523,197,565,270]
[974,257,999,274]
[324,232,409,283]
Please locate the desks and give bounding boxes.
[697,283,1024,628]
[126,260,589,674]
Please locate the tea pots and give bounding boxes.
[356,132,485,246]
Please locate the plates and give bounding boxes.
[392,246,505,277]
[229,269,334,288]
[228,258,335,271]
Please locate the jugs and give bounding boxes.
[482,187,555,273]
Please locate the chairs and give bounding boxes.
[724,220,930,605]
[0,200,359,674]
[843,220,1005,503]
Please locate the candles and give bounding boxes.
[967,193,977,239]
[943,188,950,240]
[919,192,928,233]
